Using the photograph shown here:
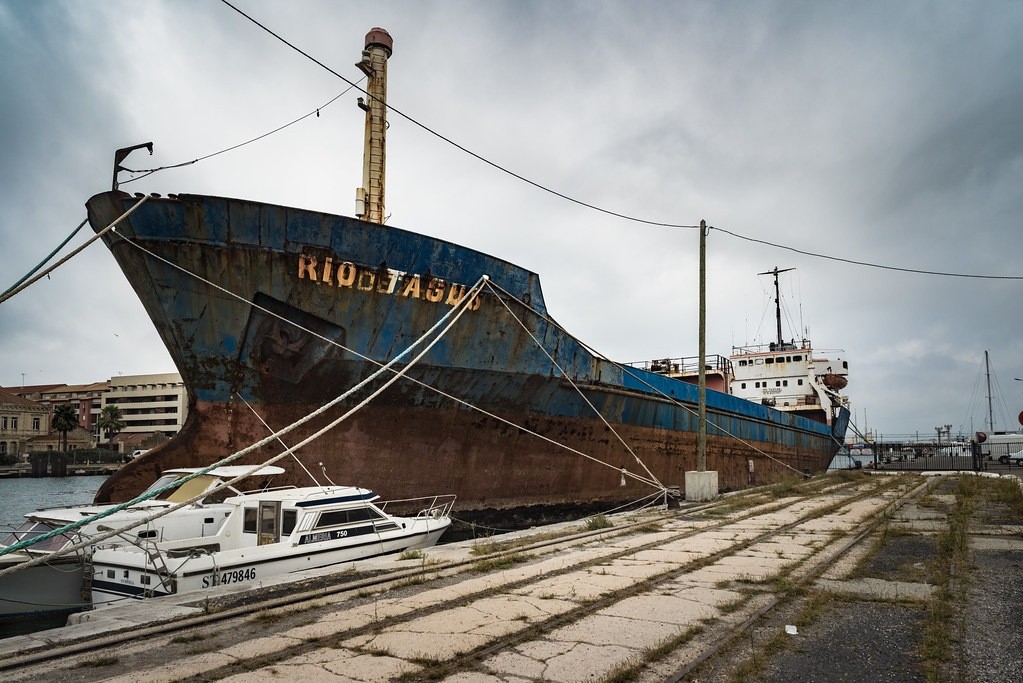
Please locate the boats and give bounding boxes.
[838,450,846,455]
[0,464,297,634]
[83,27,848,535]
[90,485,456,609]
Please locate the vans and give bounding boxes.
[981,436,990,456]
[127,450,150,460]
[989,434,1023,464]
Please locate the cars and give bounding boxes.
[1010,450,1023,466]
